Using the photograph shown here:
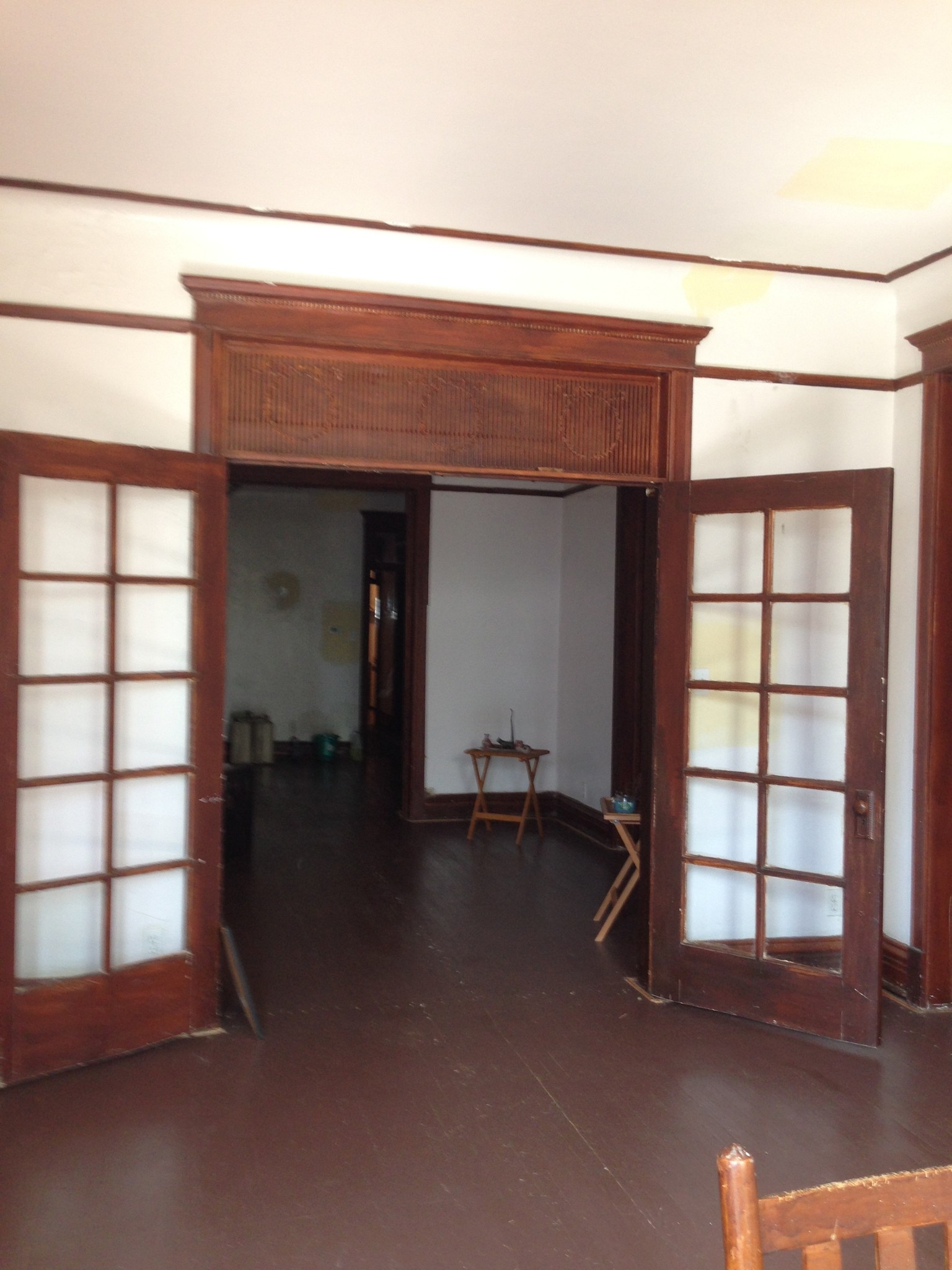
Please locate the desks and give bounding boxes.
[463,747,552,844]
[591,796,642,943]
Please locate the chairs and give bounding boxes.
[715,1141,949,1269]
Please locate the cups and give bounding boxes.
[615,791,638,813]
